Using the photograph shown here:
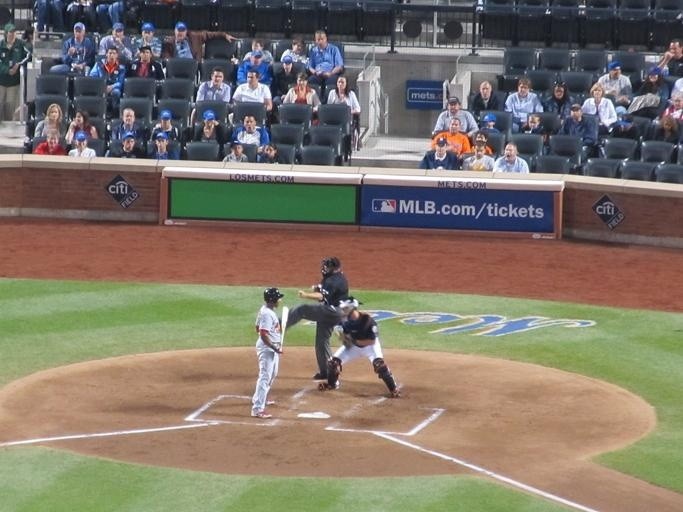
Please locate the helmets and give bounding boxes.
[320,255,340,276]
[337,298,359,317]
[263,286,284,303]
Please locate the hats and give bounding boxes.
[447,96,459,104]
[202,109,215,120]
[483,113,496,121]
[435,137,448,145]
[5,22,17,33]
[281,55,292,64]
[71,21,187,37]
[75,130,168,143]
[607,61,622,72]
[160,108,172,121]
[250,49,262,58]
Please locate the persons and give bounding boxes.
[420,37,683,174]
[318,299,401,399]
[1,1,362,163]
[251,288,283,419]
[279,256,349,378]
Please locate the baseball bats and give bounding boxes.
[278,306,291,352]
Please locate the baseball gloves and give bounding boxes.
[336,331,353,348]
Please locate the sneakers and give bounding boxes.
[259,412,272,419]
[265,399,275,405]
